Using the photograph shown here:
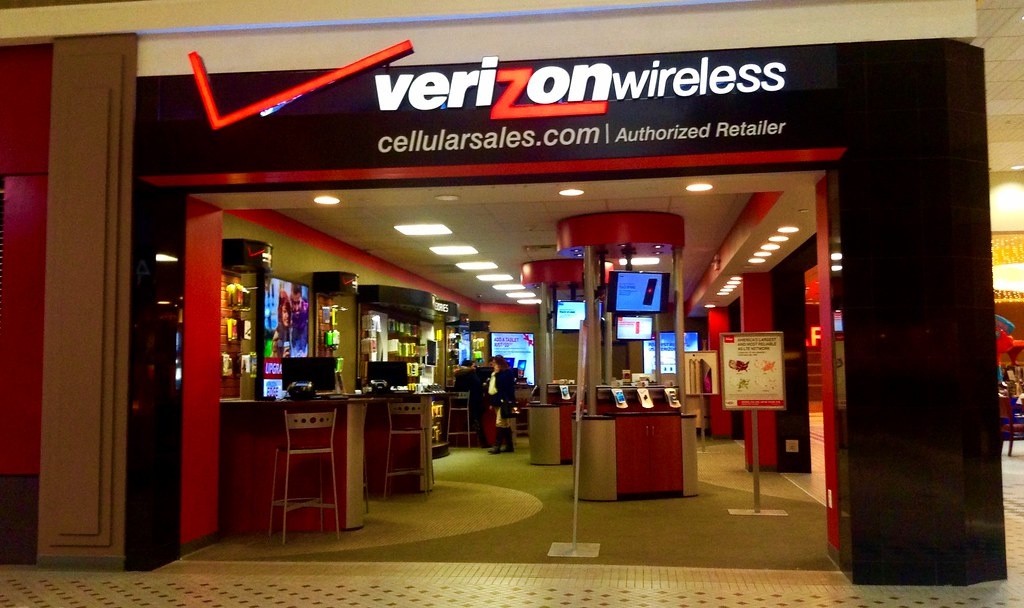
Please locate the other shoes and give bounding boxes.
[488,449,500,454]
[502,448,514,452]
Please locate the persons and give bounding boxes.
[452,354,517,454]
[272,284,309,357]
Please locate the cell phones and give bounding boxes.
[689,358,712,393]
[643,279,657,305]
[670,391,677,403]
[615,391,625,403]
[517,360,526,378]
[562,387,568,396]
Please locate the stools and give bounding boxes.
[381,402,429,497]
[269,408,340,544]
[446,391,476,448]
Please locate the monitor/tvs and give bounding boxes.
[425,338,438,367]
[281,356,336,400]
[606,270,671,314]
[475,366,495,385]
[615,315,654,341]
[554,300,587,331]
[367,361,408,386]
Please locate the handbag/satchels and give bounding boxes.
[501,401,520,418]
